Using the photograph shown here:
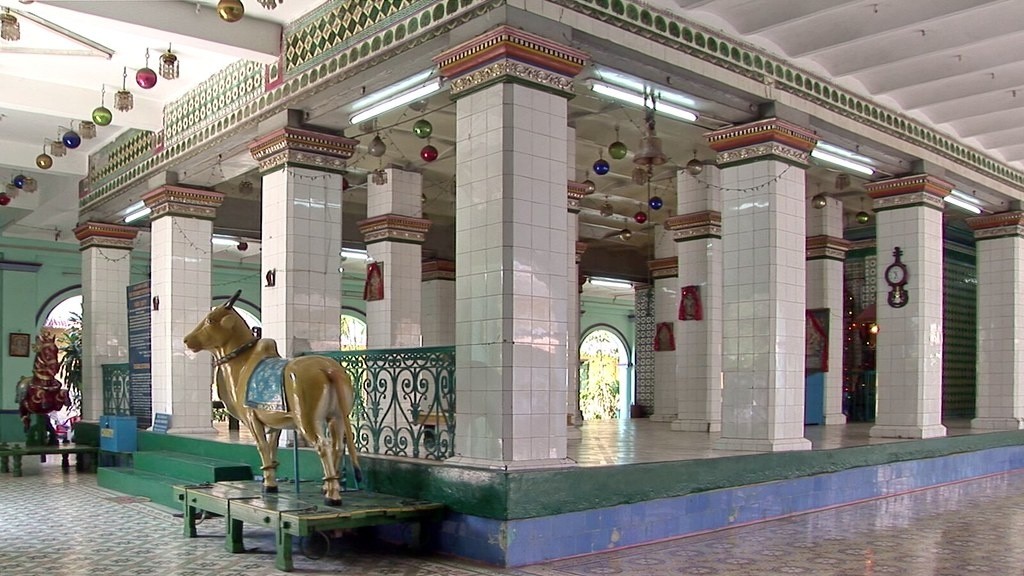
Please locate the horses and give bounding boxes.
[13,331,74,447]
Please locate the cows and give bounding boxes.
[181,287,365,507]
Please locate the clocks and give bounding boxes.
[885,245,909,308]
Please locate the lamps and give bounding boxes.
[347,77,445,125]
[113,66,134,113]
[49,125,67,158]
[587,79,701,123]
[77,119,97,140]
[834,173,852,191]
[22,173,38,194]
[811,148,877,177]
[630,139,647,186]
[238,174,254,196]
[943,195,984,215]
[122,207,153,223]
[600,195,613,218]
[371,156,388,186]
[158,43,181,80]
[5,174,20,200]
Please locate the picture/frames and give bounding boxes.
[9,332,32,358]
